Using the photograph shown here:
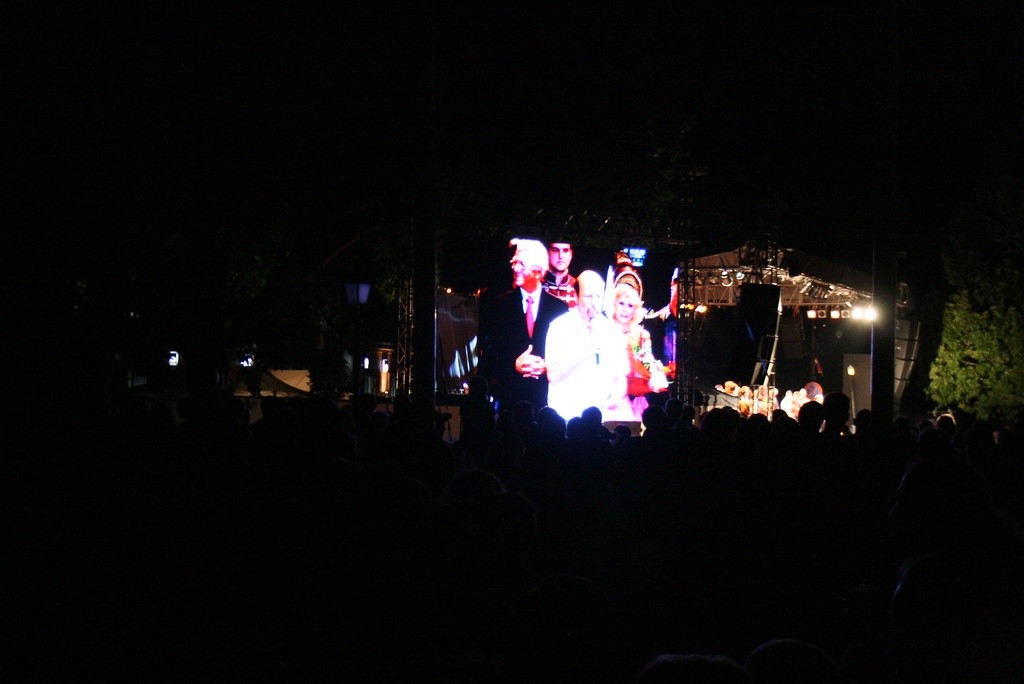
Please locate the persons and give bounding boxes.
[545,241,579,311]
[0,381,1024,684]
[480,237,568,417]
[603,250,672,421]
[546,270,630,422]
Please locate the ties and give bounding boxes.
[525,296,534,340]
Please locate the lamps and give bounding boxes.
[688,269,705,286]
[706,272,719,284]
[736,273,746,286]
[721,271,733,287]
[799,281,834,300]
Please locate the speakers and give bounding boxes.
[781,314,810,360]
[892,317,923,396]
